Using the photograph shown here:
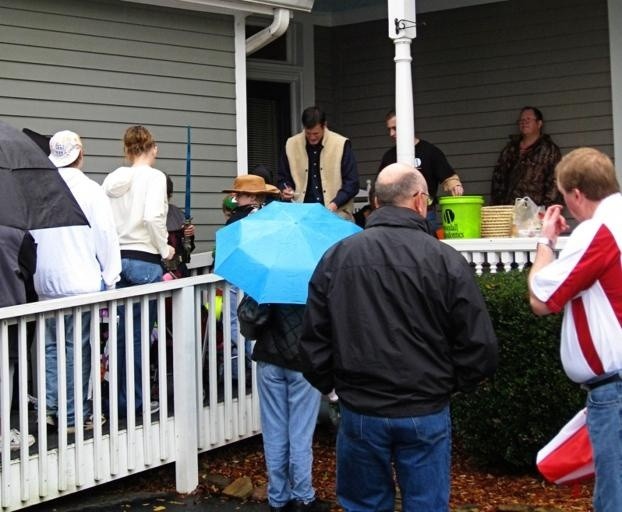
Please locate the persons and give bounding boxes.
[1,223,36,450]
[276,106,360,224]
[525,147,621,512]
[99,124,171,421]
[26,129,121,433]
[235,292,333,512]
[372,110,464,225]
[163,173,196,281]
[298,161,501,511]
[491,106,562,212]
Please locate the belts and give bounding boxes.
[581,373,622,391]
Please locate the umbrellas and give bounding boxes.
[212,199,369,306]
[0,115,93,229]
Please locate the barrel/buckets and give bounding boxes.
[438,195,485,239]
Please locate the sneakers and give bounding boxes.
[46,416,56,426]
[67,414,106,433]
[0,429,36,455]
[151,401,159,414]
[270,502,291,512]
[291,500,333,511]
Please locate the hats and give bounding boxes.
[48,130,81,168]
[223,175,281,194]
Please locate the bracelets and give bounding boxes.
[534,237,554,251]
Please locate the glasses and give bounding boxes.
[413,192,434,206]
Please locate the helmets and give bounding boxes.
[223,192,240,210]
